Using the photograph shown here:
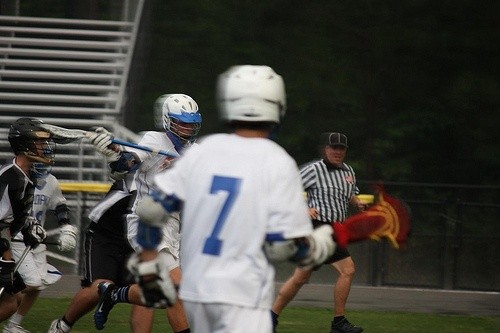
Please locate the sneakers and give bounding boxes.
[330,318,364,333]
[48,319,72,333]
[94,280,119,330]
[2,326,29,333]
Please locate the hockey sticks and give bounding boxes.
[48,124,179,160]
[0,243,32,296]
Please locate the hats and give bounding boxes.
[323,132,349,147]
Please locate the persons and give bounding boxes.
[0,93,368,333]
[137,65,336,333]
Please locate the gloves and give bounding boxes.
[89,127,122,162]
[0,256,16,292]
[21,216,46,249]
[299,225,336,271]
[134,257,177,308]
[58,225,78,253]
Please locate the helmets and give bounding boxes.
[215,65,286,122]
[8,118,56,178]
[162,94,202,140]
